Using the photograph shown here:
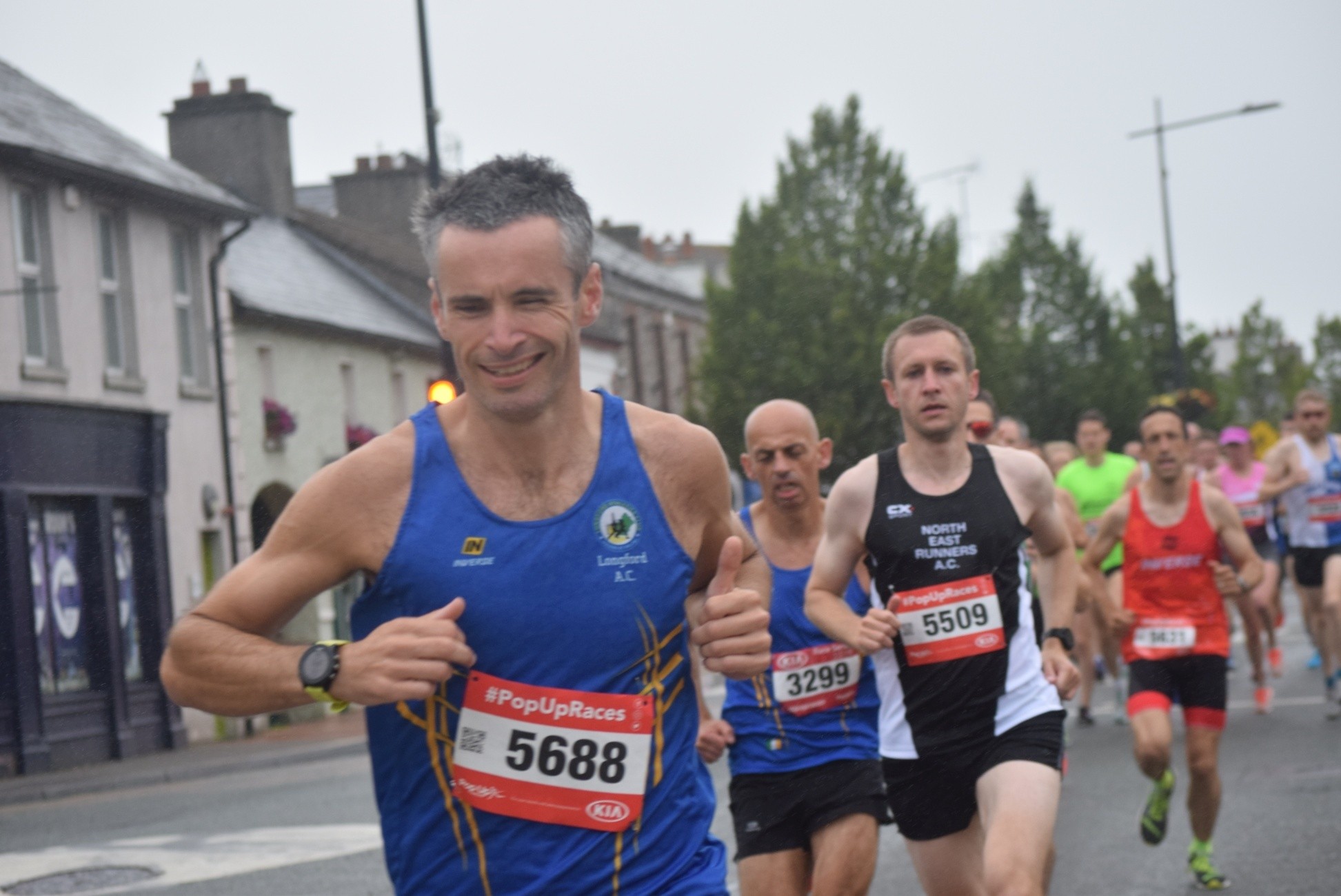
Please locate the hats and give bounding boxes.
[1219,426,1251,445]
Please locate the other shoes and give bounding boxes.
[1255,688,1271,711]
[1080,708,1094,725]
[1325,682,1341,718]
[1268,647,1282,667]
[1307,651,1322,670]
[1274,613,1284,628]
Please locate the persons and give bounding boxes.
[160,154,772,895]
[966,389,1341,889]
[804,316,1079,896]
[688,399,894,895]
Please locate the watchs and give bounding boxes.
[298,639,352,712]
[1042,626,1074,650]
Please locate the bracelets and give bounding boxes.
[1236,575,1248,593]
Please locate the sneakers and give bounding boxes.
[1187,839,1231,891]
[1141,769,1176,844]
